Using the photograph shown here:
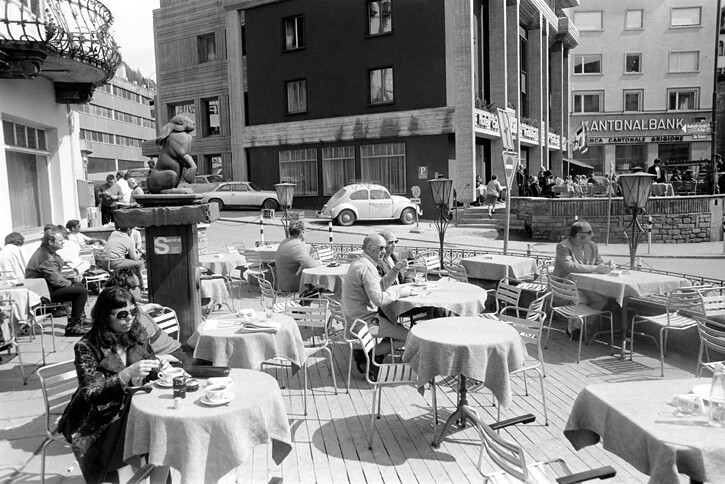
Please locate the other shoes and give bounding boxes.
[491,211,495,214]
[488,215,492,219]
[353,349,372,373]
[566,328,584,341]
[367,348,387,382]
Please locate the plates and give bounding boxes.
[156,379,174,387]
[409,283,427,286]
[199,395,235,406]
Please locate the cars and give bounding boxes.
[314,183,423,226]
[188,174,279,211]
[125,167,152,193]
[589,176,623,197]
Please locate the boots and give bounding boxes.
[82,315,94,325]
[65,318,91,335]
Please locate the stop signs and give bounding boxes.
[505,156,514,177]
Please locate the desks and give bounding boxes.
[298,264,351,298]
[62,256,94,306]
[185,313,307,376]
[382,280,489,328]
[245,243,311,268]
[348,250,399,262]
[651,183,674,196]
[0,289,42,356]
[563,376,725,484]
[560,269,691,355]
[198,253,249,281]
[122,368,292,484]
[400,317,529,446]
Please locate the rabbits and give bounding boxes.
[144,113,199,197]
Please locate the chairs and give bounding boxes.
[0,240,725,484]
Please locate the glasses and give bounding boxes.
[110,307,138,319]
[576,231,591,235]
[369,245,388,250]
[386,240,398,244]
[130,284,142,291]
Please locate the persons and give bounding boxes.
[485,174,503,219]
[276,222,323,318]
[475,153,725,206]
[0,171,213,365]
[57,287,173,484]
[341,230,410,382]
[553,221,615,340]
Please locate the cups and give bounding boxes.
[158,367,184,382]
[205,384,228,403]
[677,392,695,416]
[414,276,425,284]
[264,303,273,318]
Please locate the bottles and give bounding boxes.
[707,362,725,428]
